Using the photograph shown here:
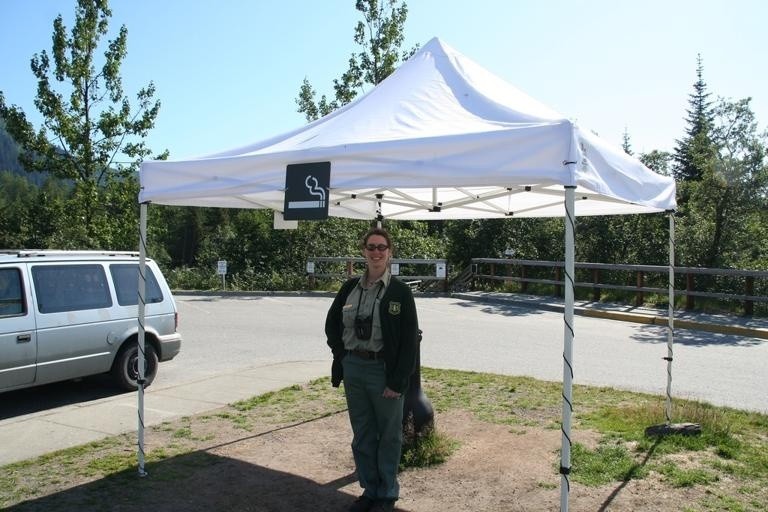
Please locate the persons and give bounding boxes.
[324,230,419,512]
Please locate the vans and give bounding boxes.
[0,243,186,396]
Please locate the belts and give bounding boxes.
[349,350,382,359]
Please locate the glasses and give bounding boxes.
[366,243,388,251]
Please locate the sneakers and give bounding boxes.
[351,496,396,511]
